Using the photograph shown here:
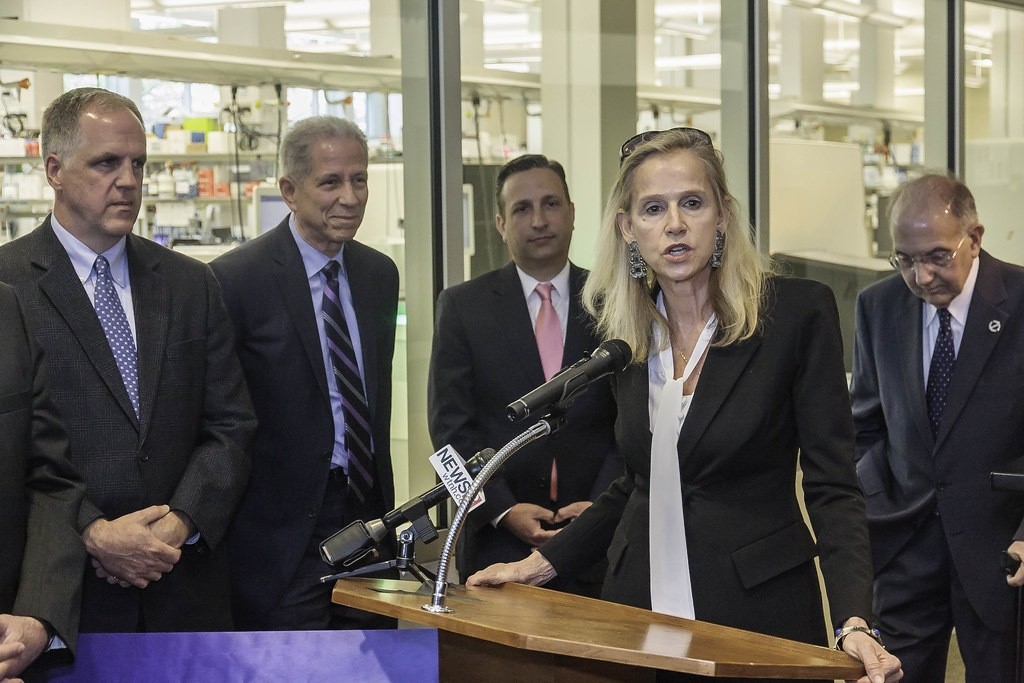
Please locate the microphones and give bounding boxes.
[317,447,505,566]
[506,339,633,423]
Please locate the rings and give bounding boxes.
[113,577,118,582]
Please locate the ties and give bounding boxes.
[90,255,141,423]
[318,261,376,514]
[926,307,956,437]
[530,283,565,505]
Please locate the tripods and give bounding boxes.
[320,497,449,595]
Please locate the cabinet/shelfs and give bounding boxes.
[0,153,273,261]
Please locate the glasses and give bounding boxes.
[887,223,976,273]
[618,127,714,175]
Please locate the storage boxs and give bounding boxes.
[0,116,239,200]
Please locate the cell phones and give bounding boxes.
[1000,551,1021,577]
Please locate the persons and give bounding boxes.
[465,128,904,683]
[427,154,612,587]
[848,175,1024,683]
[0,281,88,683]
[0,88,258,634]
[207,117,400,631]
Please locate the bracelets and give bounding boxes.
[833,626,885,651]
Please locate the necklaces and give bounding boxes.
[672,336,701,375]
